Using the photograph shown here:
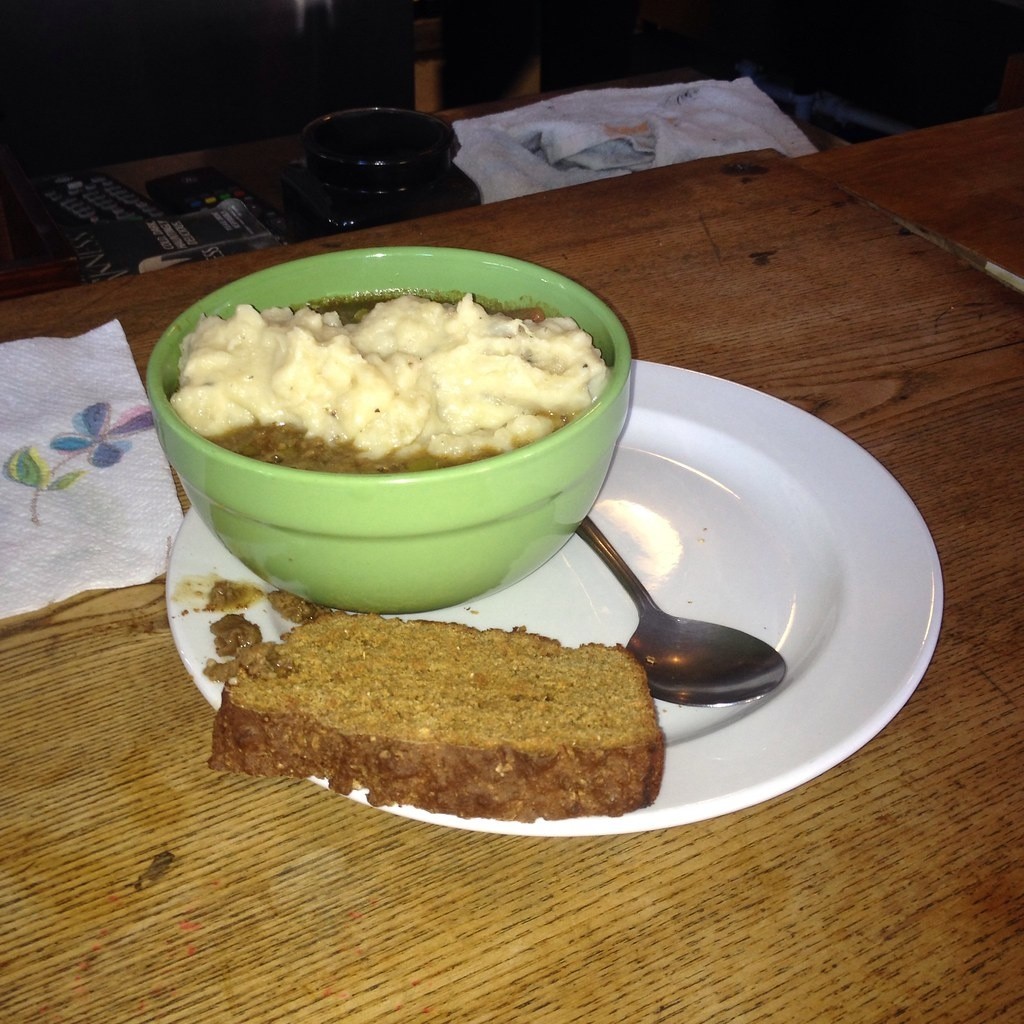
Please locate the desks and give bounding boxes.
[109,75,671,218]
[0,147,1024,1024]
[787,108,1024,298]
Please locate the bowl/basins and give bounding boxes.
[147,245,633,617]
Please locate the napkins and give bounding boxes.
[0,318,184,620]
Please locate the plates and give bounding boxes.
[164,366,944,838]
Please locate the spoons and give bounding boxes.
[576,516,789,706]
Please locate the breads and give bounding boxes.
[208,611,666,824]
